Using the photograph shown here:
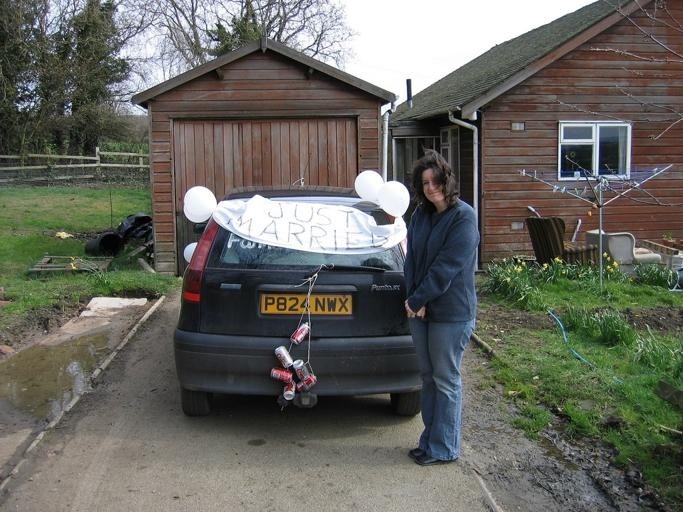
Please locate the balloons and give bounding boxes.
[352,169,383,205]
[184,185,218,210]
[182,242,198,262]
[377,180,411,218]
[183,196,211,224]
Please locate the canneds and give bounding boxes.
[293,359,309,381]
[296,374,317,393]
[283,381,296,401]
[270,367,293,384]
[274,345,293,368]
[290,322,309,345]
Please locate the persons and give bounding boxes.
[401,149,480,466]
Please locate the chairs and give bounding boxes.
[525,216,661,275]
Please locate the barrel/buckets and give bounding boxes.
[84,230,125,258]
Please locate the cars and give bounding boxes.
[173,183,421,416]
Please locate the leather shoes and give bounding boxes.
[416,455,444,464]
[408,448,426,457]
